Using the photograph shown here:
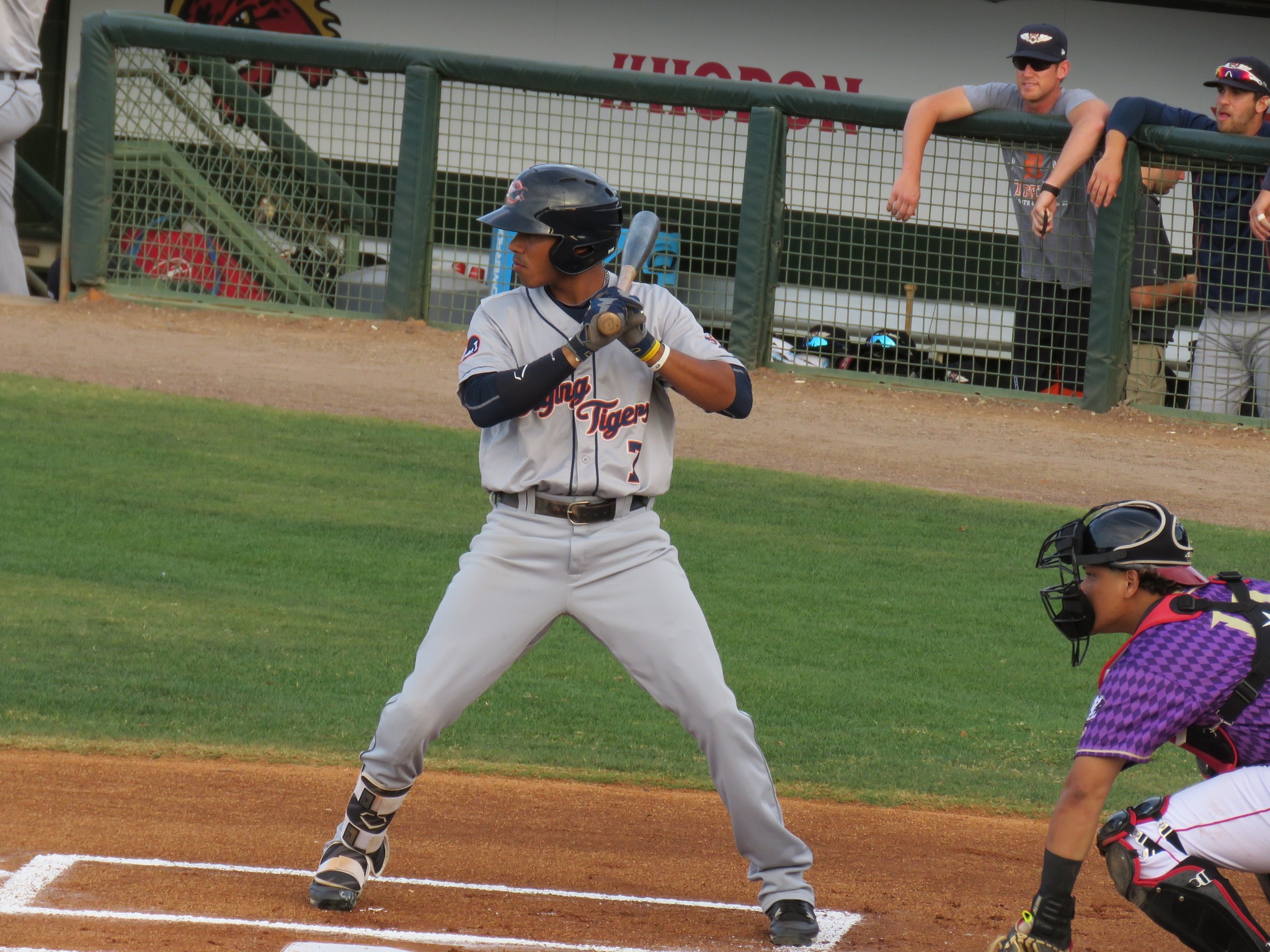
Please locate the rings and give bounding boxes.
[1256,213,1266,221]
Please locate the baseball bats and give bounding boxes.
[905,283,918,336]
[597,210,663,337]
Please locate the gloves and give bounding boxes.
[591,287,656,359]
[566,298,647,362]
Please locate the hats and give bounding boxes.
[1006,23,1067,62]
[790,325,850,354]
[1203,56,1270,97]
[861,329,916,363]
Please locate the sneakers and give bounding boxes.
[766,899,819,946]
[310,843,371,911]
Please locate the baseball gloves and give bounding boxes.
[995,916,1073,952]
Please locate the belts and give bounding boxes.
[496,491,649,525]
[0,71,34,80]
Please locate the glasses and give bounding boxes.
[1215,65,1270,93]
[802,337,850,349]
[864,334,902,348]
[1012,55,1059,71]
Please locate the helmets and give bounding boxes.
[1083,504,1210,585]
[476,163,623,274]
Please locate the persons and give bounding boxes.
[986,496,1270,952]
[306,161,821,945]
[1086,56,1270,421]
[886,22,1115,398]
[0,0,108,301]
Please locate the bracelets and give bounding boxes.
[1040,183,1061,199]
[639,340,671,371]
